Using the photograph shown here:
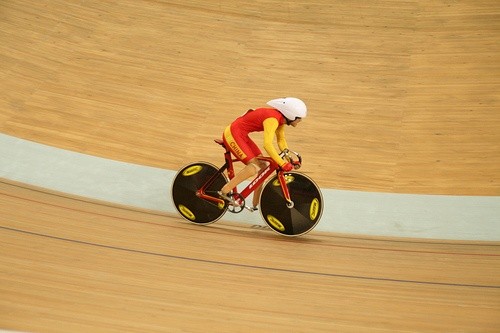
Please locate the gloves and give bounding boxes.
[293,160,302,169]
[281,162,293,171]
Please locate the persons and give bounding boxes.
[220,97,308,210]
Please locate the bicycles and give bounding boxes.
[172,139,324,237]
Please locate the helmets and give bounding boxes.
[265,96,307,120]
